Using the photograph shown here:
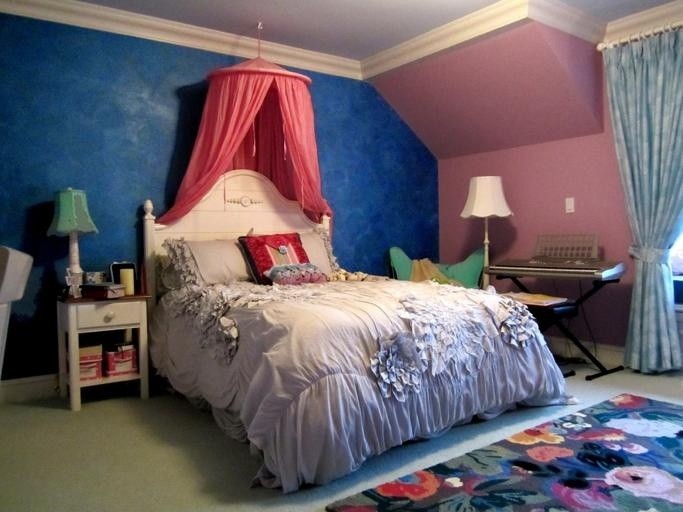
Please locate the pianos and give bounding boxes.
[483,255,625,281]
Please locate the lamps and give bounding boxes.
[48,187,100,295]
[459,175,514,288]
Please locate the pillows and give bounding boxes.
[161,227,339,291]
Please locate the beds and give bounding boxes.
[139,169,579,491]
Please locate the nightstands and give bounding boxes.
[56,296,153,413]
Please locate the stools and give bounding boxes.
[502,290,578,379]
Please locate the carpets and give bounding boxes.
[325,394,683,512]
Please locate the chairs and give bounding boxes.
[389,247,484,288]
